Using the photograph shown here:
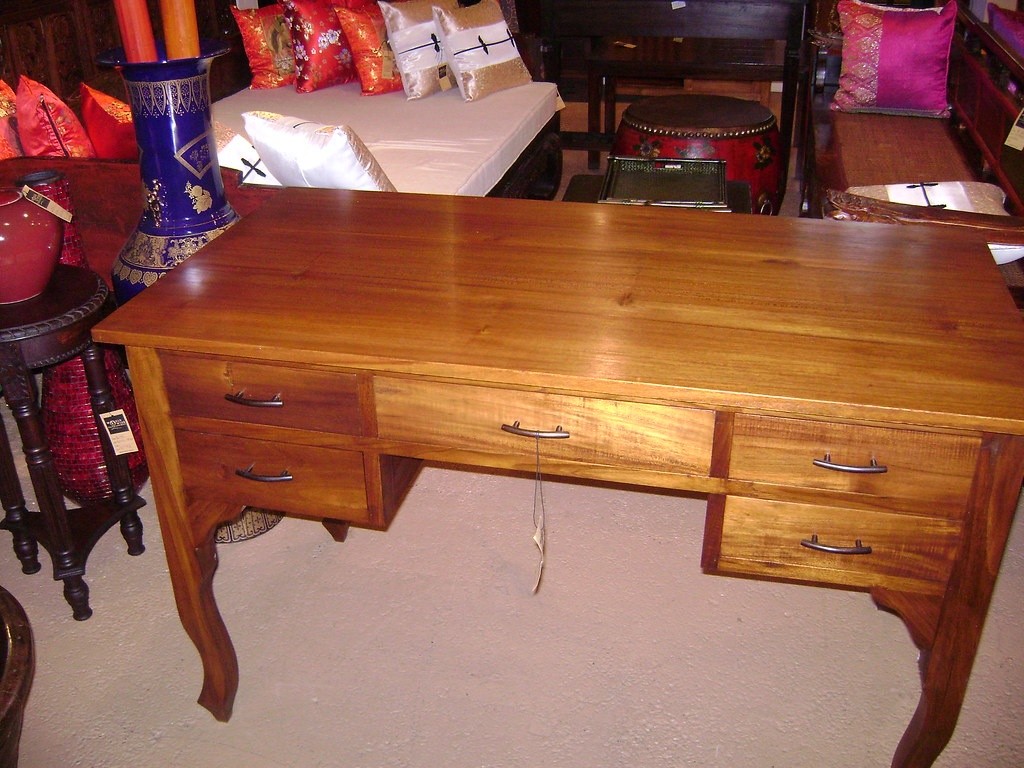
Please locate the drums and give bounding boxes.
[608,92,786,217]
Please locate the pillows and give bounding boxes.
[80,82,138,160]
[230,4,295,90]
[278,0,375,95]
[334,0,410,96]
[246,111,398,192]
[431,0,532,104]
[16,74,95,158]
[829,0,957,118]
[0,79,24,160]
[378,0,460,100]
[212,120,282,186]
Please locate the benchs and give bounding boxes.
[795,0,1024,311]
[0,0,283,368]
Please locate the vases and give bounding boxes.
[94,36,286,543]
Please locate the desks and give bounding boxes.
[0,264,147,621]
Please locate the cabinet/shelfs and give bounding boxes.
[91,186,1024,768]
